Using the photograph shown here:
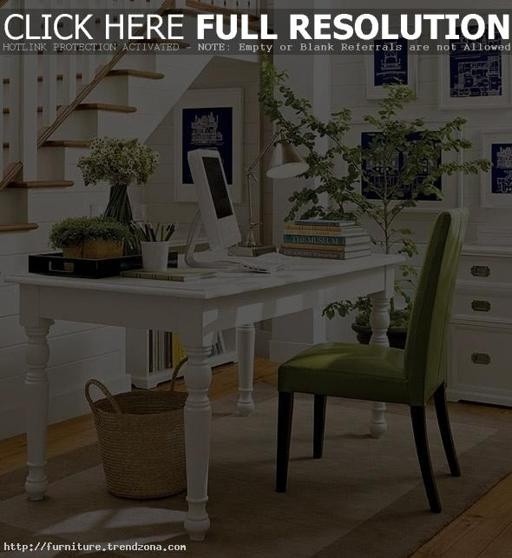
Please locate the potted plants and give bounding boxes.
[48,212,127,260]
[250,48,493,353]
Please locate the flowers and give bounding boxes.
[78,132,164,185]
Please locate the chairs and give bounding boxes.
[269,207,479,511]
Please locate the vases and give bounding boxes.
[104,177,136,226]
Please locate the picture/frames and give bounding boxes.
[170,87,245,204]
[436,21,512,110]
[361,27,418,101]
[482,126,511,209]
[344,121,464,211]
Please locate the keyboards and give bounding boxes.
[244,254,285,273]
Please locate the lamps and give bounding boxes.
[226,115,311,256]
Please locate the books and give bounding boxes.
[278,218,372,260]
[119,267,221,282]
[150,329,226,372]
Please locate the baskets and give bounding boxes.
[85,357,186,502]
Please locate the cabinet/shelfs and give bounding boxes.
[417,243,511,410]
[121,236,238,389]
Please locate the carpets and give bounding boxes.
[2,381,510,558]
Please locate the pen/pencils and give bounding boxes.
[130,220,176,242]
[244,265,268,271]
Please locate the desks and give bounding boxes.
[3,247,416,546]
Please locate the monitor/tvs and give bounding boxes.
[185,149,241,268]
